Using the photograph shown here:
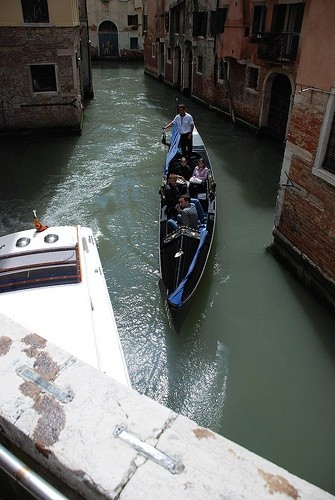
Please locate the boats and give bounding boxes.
[158,125,217,312]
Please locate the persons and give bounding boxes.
[189,158,208,199]
[176,157,192,181]
[167,195,198,234]
[163,103,195,157]
[164,173,206,223]
